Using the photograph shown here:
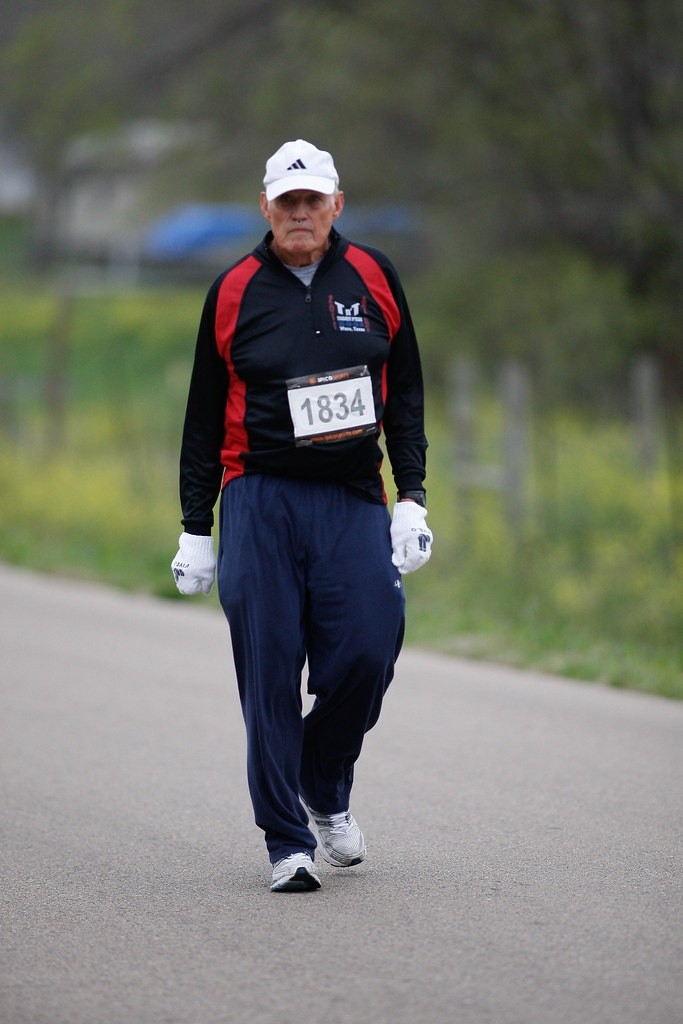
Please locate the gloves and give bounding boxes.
[389,503,433,576]
[171,533,216,597]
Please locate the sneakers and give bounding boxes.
[300,796,367,867]
[270,852,321,892]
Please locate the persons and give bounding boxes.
[167,137,436,894]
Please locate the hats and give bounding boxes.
[264,138,340,200]
